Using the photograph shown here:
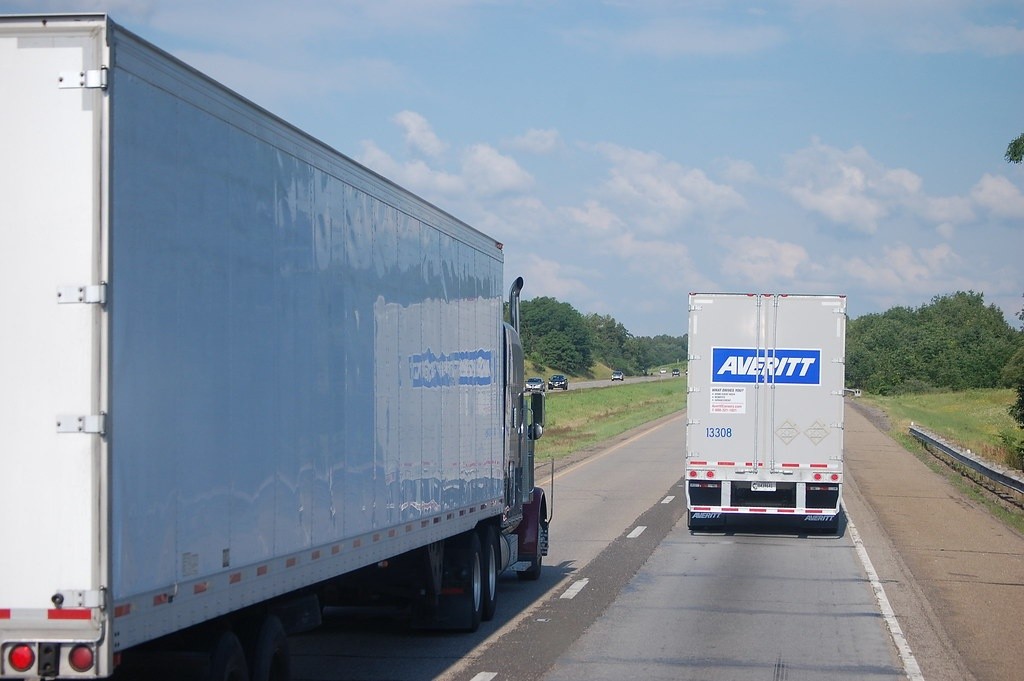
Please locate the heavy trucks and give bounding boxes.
[0,10,557,681]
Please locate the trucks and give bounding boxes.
[684,290,847,528]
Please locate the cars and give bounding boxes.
[524,378,545,393]
[612,371,624,381]
[672,368,681,376]
[660,369,666,374]
[548,375,568,391]
